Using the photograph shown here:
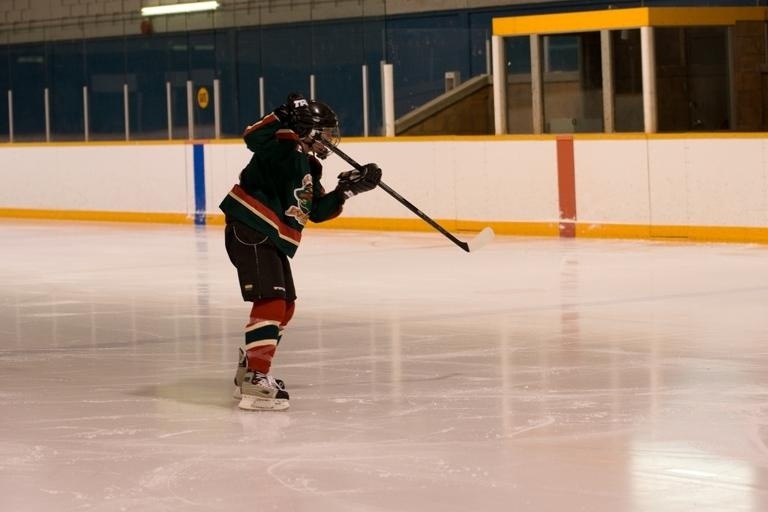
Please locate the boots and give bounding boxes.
[241,371,291,401]
[233,344,287,390]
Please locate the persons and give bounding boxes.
[218,94,382,400]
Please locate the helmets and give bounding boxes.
[296,99,342,160]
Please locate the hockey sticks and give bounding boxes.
[306,128,493,253]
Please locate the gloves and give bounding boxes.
[278,91,314,138]
[337,162,383,200]
